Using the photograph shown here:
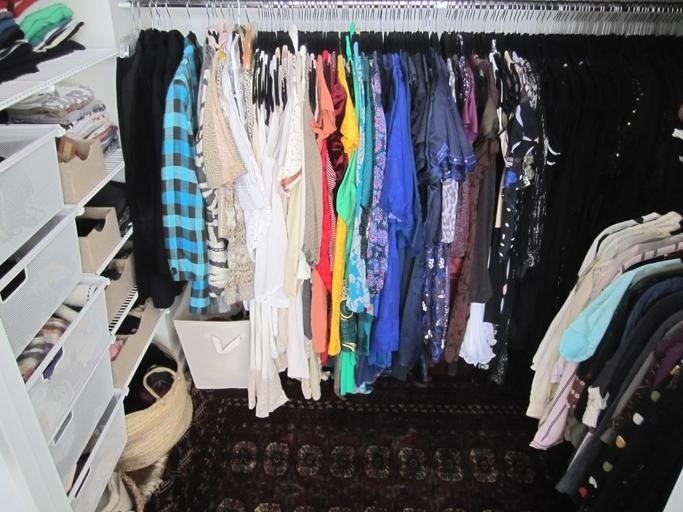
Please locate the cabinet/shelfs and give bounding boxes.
[0,1,166,511]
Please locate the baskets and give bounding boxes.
[112,339,194,472]
